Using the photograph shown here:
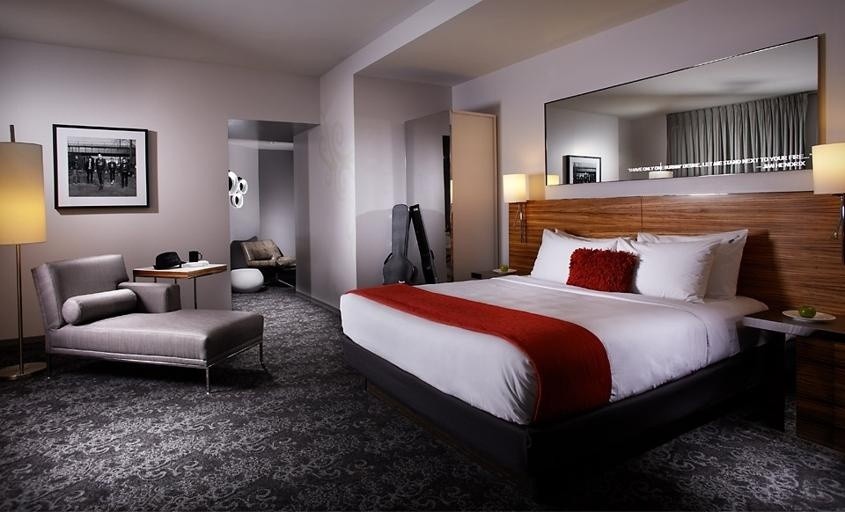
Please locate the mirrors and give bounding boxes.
[544,35,819,186]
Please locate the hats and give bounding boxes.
[153,252,186,269]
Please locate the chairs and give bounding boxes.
[230,235,296,289]
[32,253,266,395]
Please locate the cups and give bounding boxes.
[189,250,203,261]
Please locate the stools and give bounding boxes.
[231,268,264,292]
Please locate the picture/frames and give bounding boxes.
[51,123,150,209]
[564,155,601,183]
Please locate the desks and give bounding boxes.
[134,263,227,310]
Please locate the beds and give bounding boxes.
[324,275,783,504]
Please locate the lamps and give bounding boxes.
[1,124,50,381]
[547,175,559,185]
[648,162,674,179]
[812,144,845,264]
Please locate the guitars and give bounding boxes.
[409,205,440,284]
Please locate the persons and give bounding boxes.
[108,158,116,184]
[575,172,596,183]
[96,153,106,190]
[71,155,82,183]
[120,158,129,188]
[86,157,94,183]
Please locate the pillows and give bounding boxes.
[615,237,722,304]
[566,248,637,294]
[636,227,750,298]
[62,289,137,325]
[554,228,636,244]
[529,228,617,287]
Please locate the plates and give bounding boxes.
[492,268,517,275]
[781,310,837,324]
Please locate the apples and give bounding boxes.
[500,265,508,271]
[800,306,816,317]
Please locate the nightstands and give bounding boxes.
[740,307,845,435]
[471,270,528,281]
